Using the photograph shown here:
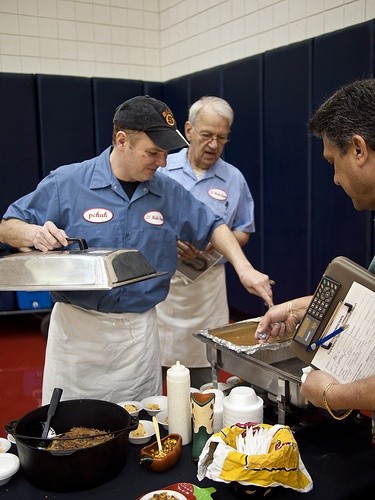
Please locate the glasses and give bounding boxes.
[194,125,231,144]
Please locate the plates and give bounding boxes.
[140,483,216,500]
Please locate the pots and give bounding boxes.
[3,399,139,493]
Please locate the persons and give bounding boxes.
[256,78,375,500]
[155,97,255,390]
[0,95,274,405]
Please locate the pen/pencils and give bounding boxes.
[304,321,353,355]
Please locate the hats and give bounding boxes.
[113,95,190,150]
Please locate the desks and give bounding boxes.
[0,413,375,500]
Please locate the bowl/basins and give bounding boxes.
[116,376,240,444]
[0,453,20,486]
[140,433,182,471]
[0,433,16,453]
[202,386,264,434]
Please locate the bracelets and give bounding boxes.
[288,301,292,313]
[323,382,352,420]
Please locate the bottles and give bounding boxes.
[166,361,192,445]
